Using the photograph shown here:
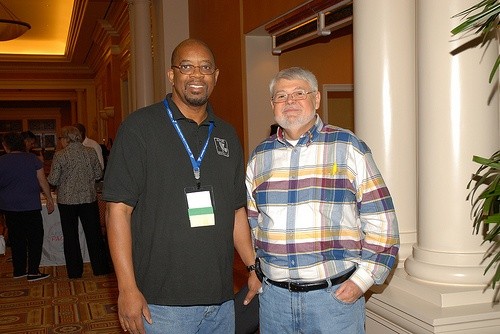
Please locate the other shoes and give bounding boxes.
[27,273,50,281]
[13,271,28,278]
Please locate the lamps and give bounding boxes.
[0,0,31,41]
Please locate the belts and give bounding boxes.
[256,257,356,292]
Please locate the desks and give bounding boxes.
[38,192,91,266]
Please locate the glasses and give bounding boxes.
[271,89,315,103]
[171,62,217,75]
[58,137,65,140]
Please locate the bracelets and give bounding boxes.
[246,264,255,272]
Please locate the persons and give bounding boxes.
[0,123,109,282]
[101,38,261,334]
[245,67,400,334]
